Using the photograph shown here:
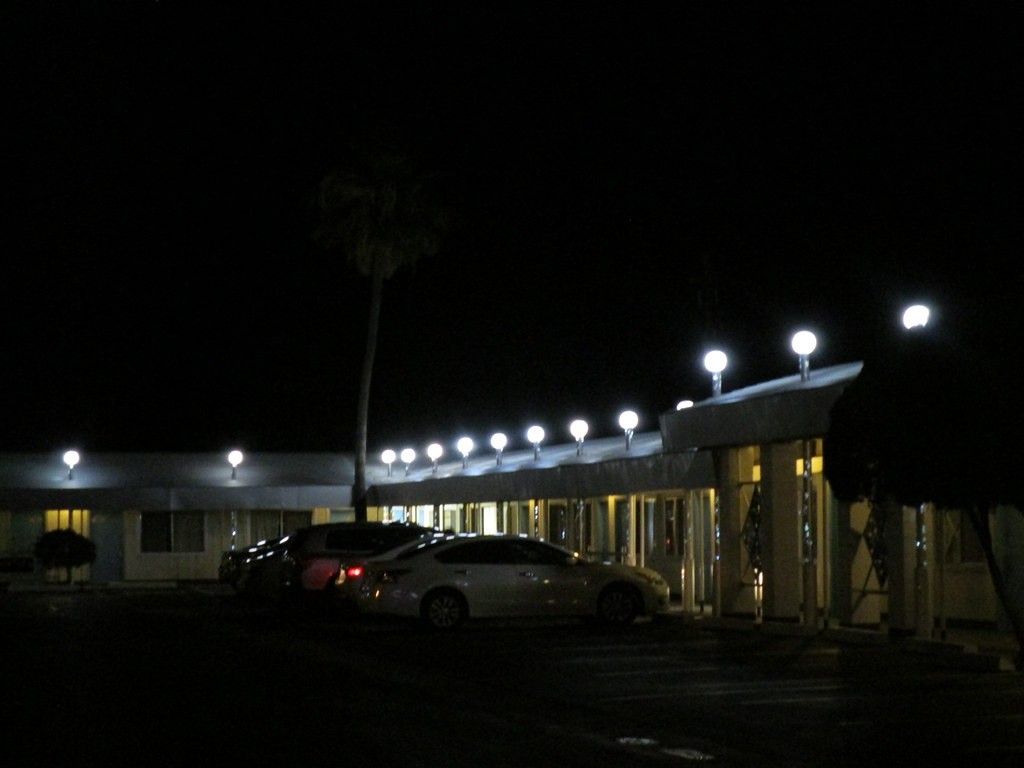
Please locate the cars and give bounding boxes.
[359,536,669,631]
[219,521,469,613]
